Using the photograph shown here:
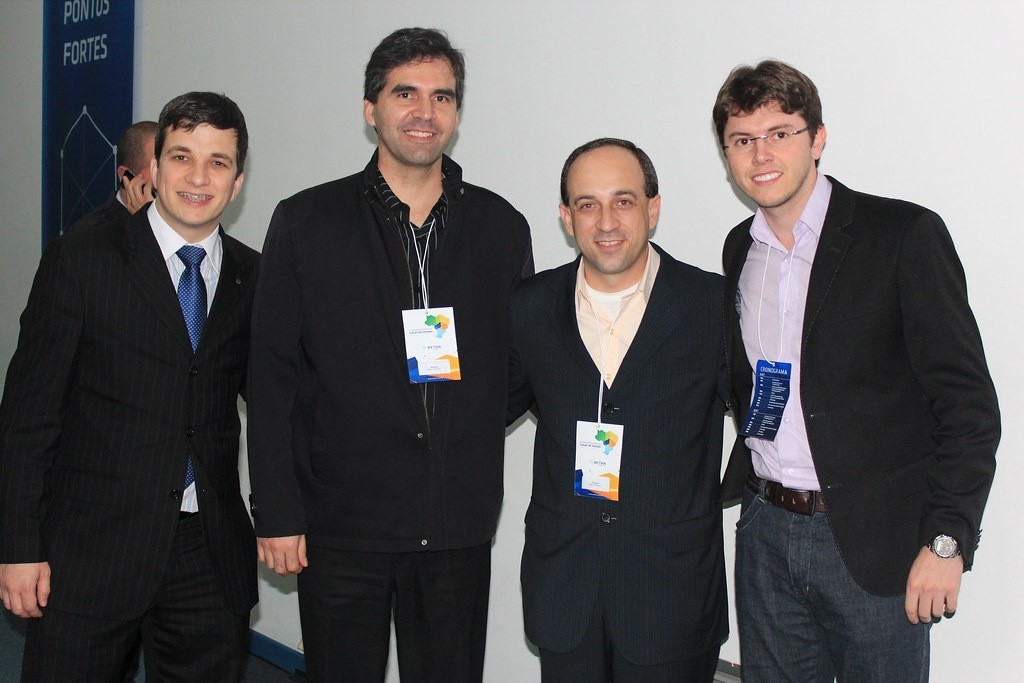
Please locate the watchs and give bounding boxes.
[925,533,963,560]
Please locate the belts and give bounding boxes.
[744,468,827,518]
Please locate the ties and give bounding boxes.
[174,247,209,491]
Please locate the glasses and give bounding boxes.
[722,123,811,156]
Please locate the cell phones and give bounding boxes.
[120,169,157,198]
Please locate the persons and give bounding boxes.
[248,28,537,683]
[43,121,164,276]
[0,92,265,683]
[505,139,756,682]
[711,58,1002,683]
